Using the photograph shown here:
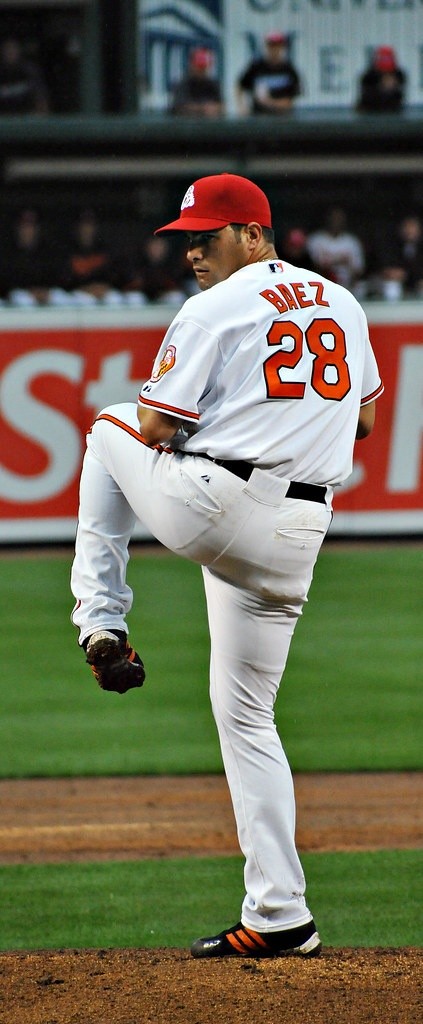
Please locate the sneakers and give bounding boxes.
[191,923,322,960]
[82,627,146,694]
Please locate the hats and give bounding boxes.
[375,45,397,73]
[152,173,272,237]
[263,34,286,44]
[192,49,212,68]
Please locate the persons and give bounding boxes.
[70,174,384,958]
[0,32,423,301]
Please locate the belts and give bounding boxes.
[202,453,326,505]
